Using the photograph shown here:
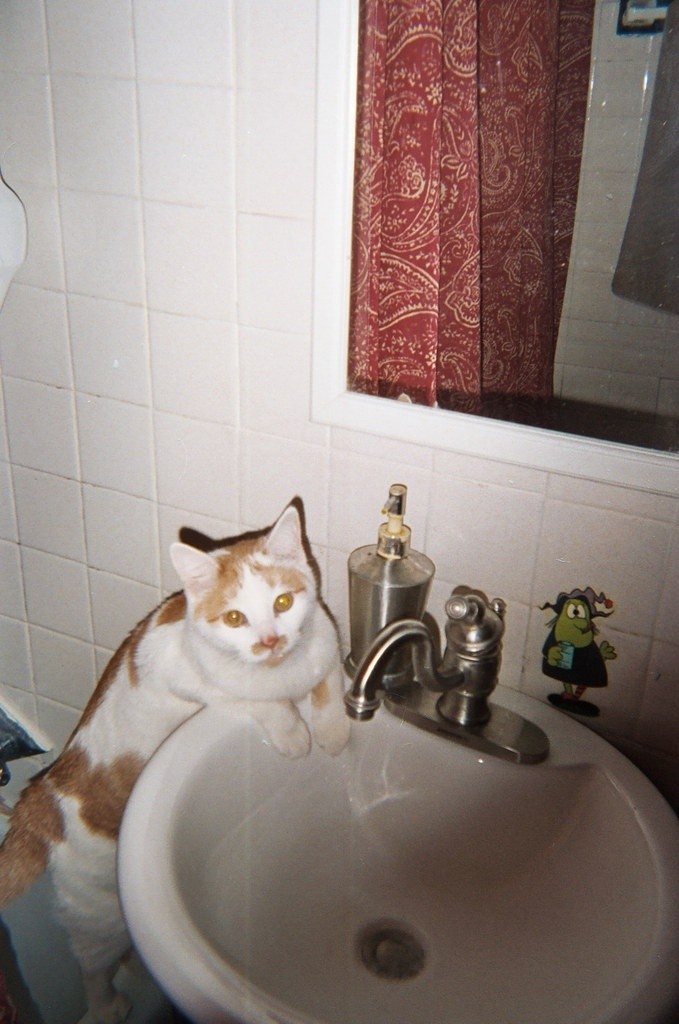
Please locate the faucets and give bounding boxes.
[342,592,507,734]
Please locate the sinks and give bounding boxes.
[117,666,678,1024]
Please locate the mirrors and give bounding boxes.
[346,0,679,461]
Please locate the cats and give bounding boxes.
[1,501,352,1023]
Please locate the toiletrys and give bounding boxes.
[341,480,436,689]
